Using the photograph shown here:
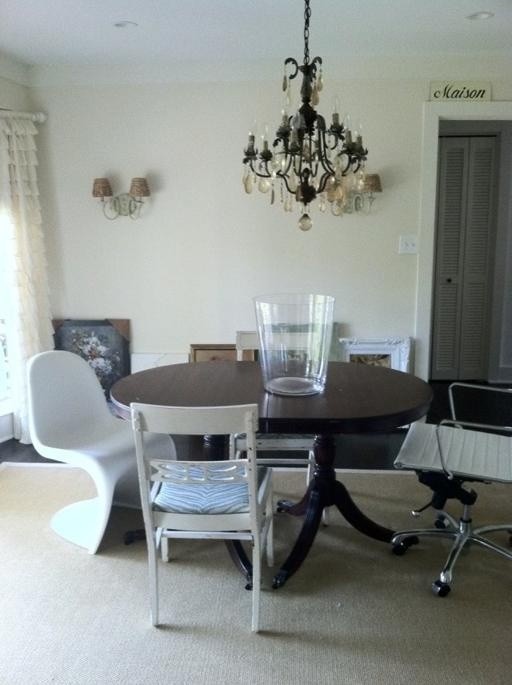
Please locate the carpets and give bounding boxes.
[0,459,511,685]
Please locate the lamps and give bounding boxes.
[240,0,383,232]
[91,176,151,222]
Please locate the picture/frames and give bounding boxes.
[51,317,132,404]
